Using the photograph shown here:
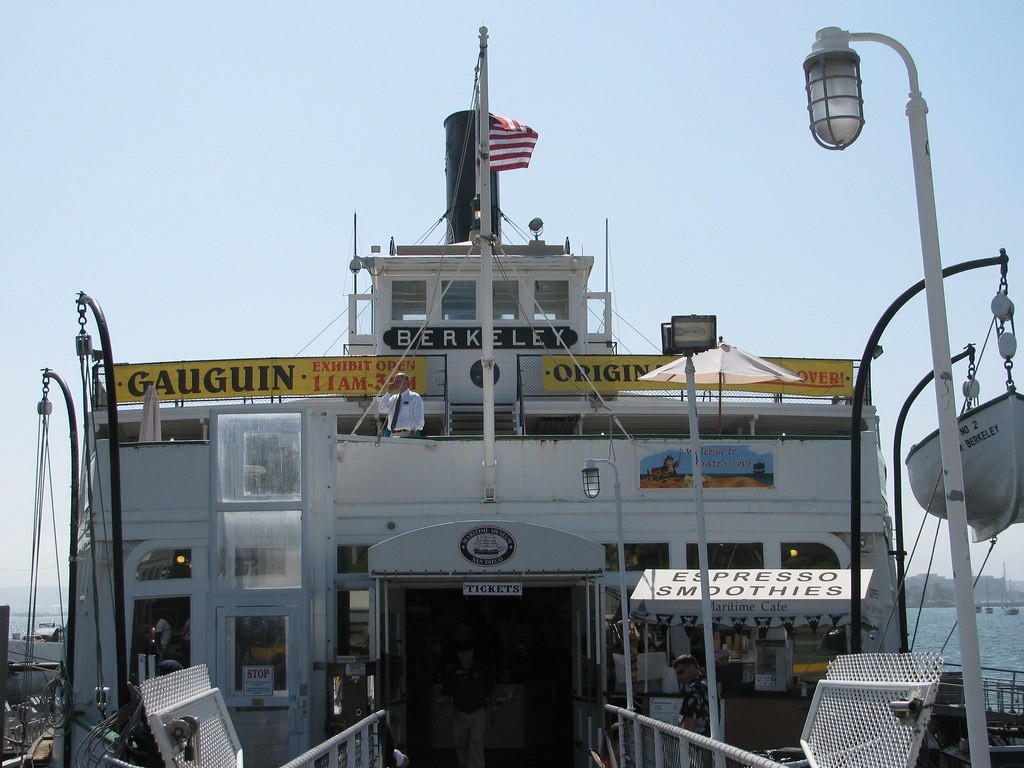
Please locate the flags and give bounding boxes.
[489,112,538,172]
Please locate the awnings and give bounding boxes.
[630,569,886,631]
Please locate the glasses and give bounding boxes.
[393,380,403,384]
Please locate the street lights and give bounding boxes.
[800,23,989,768]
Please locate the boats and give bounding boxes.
[974,605,981,613]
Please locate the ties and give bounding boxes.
[391,394,401,431]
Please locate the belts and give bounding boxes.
[390,429,409,433]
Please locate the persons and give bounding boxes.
[674,653,735,768]
[408,640,445,751]
[447,642,486,768]
[438,618,493,677]
[138,607,190,677]
[599,722,619,768]
[377,373,425,438]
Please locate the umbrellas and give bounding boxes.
[138,381,161,441]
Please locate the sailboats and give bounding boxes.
[999,562,1023,615]
[984,575,994,615]
[5,620,69,711]
